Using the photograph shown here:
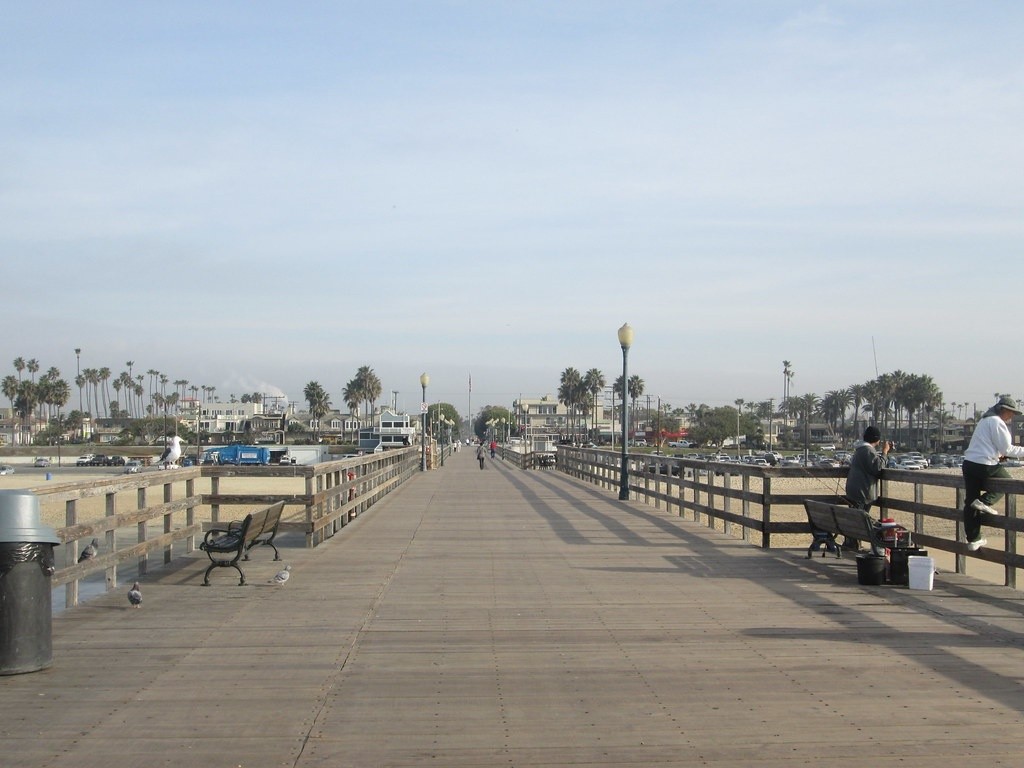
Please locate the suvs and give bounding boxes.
[124,460,142,474]
[632,439,648,448]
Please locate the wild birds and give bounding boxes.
[154,436,184,469]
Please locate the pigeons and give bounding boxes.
[128,581,143,608]
[78,536,99,563]
[266,565,291,585]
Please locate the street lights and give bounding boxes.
[440,412,445,465]
[618,321,635,501]
[57,405,61,466]
[445,418,456,457]
[521,402,529,470]
[501,417,506,460]
[169,412,178,436]
[419,371,431,471]
[486,416,499,445]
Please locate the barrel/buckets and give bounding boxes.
[890,548,928,584]
[856,553,886,585]
[907,555,935,591]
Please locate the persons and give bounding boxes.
[476,442,487,470]
[453,440,462,452]
[490,440,497,459]
[466,438,469,446]
[962,397,1024,552]
[842,426,890,556]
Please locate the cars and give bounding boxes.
[0,465,15,477]
[34,458,51,468]
[820,444,836,451]
[77,453,129,467]
[643,449,965,480]
[159,461,180,471]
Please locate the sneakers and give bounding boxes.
[967,537,987,551]
[970,498,999,516]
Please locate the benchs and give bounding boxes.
[199,498,287,588]
[537,454,553,470]
[805,497,909,562]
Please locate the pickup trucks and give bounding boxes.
[667,440,694,449]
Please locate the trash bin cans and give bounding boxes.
[0,489,60,674]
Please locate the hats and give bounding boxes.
[994,397,1022,415]
[863,426,882,444]
